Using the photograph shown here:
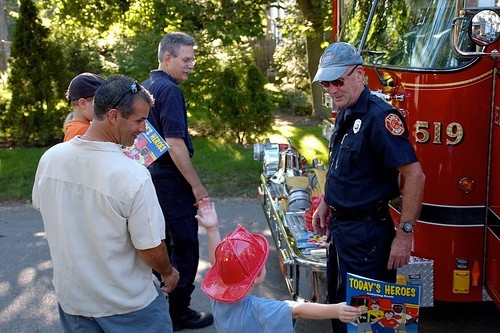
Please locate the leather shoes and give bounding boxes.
[171,310,214,331]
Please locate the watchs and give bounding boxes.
[399,221,414,233]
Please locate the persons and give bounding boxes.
[195,197,361,333]
[63,72,106,142]
[137,32,214,332]
[32,74,180,333]
[312,41,425,333]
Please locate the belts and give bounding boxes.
[327,201,388,222]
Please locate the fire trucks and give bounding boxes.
[254,0,500,312]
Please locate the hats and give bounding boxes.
[312,42,364,83]
[67,72,106,100]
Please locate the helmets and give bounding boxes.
[201,224,268,302]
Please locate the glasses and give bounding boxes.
[107,80,142,118]
[170,54,195,64]
[319,64,358,87]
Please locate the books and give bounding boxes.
[118,119,170,167]
